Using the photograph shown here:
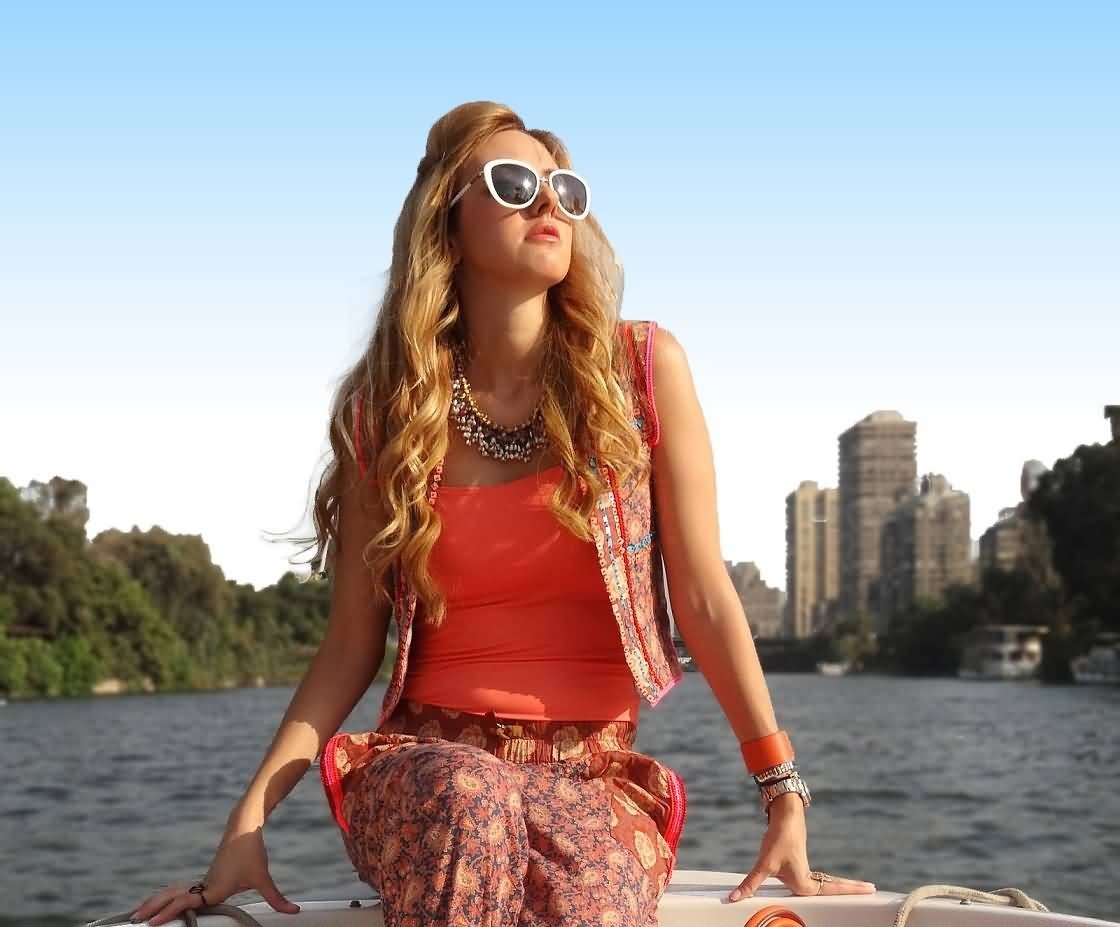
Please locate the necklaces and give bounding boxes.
[445,329,557,465]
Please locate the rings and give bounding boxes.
[810,870,833,896]
[188,882,208,909]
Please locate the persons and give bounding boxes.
[127,102,875,925]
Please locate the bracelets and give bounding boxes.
[737,729,800,792]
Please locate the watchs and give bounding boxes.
[762,776,812,818]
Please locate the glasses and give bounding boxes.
[449,159,591,221]
